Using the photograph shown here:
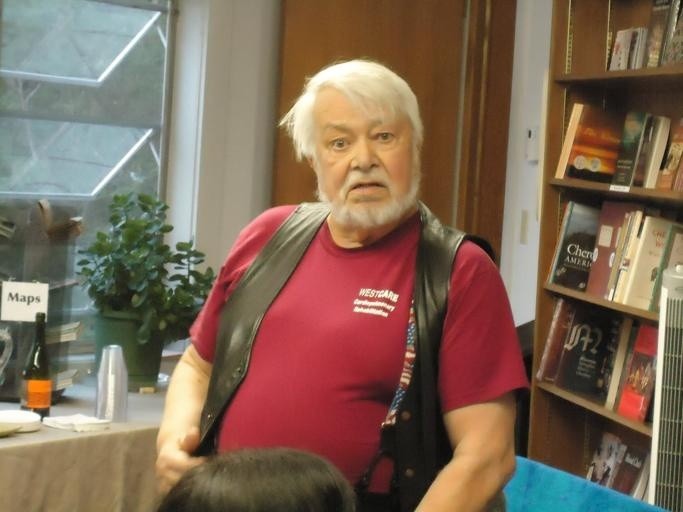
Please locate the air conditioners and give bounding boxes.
[645,260,683,512]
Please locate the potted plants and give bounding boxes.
[79,188,227,398]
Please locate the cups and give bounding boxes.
[95,344,129,421]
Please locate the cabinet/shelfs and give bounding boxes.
[525,1,683,511]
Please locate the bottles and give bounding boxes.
[22,312,52,417]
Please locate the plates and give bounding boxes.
[0,409,41,432]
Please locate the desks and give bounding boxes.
[0,354,204,512]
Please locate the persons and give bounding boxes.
[156,447,362,512]
[153,61,530,512]
